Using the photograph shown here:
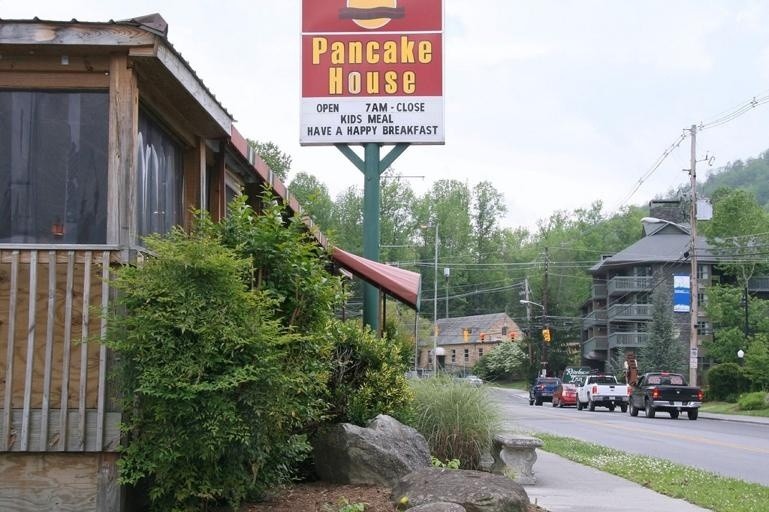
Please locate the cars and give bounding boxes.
[466,375,483,385]
[529,376,576,406]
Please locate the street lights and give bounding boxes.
[444,268,451,318]
[640,216,697,386]
[519,299,548,376]
[737,350,745,367]
[421,223,441,377]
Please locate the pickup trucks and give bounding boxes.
[576,372,703,420]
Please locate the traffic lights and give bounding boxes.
[480,333,485,342]
[509,333,514,343]
[542,330,550,342]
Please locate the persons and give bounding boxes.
[623,351,639,387]
